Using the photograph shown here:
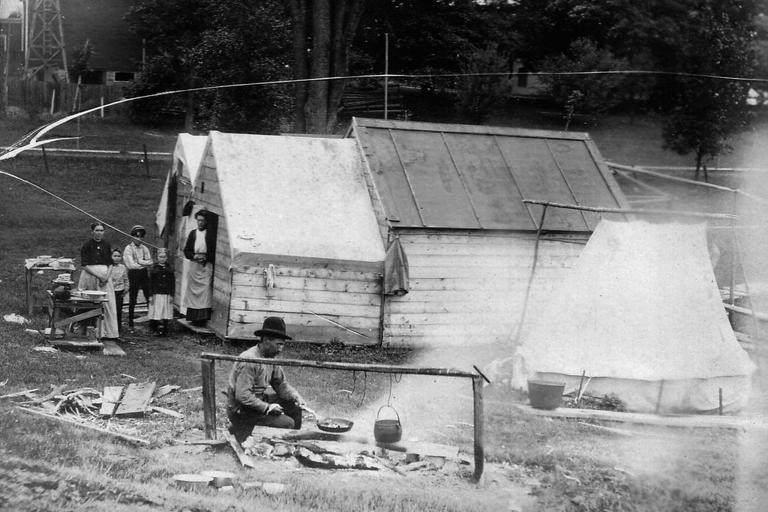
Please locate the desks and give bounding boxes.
[46,290,108,343]
[25,262,76,318]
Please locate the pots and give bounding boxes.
[293,401,354,433]
[373,404,402,444]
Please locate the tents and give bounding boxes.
[512,216,756,414]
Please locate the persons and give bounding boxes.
[226,316,306,452]
[122,224,154,331]
[145,246,175,336]
[77,221,119,339]
[108,249,130,332]
[181,209,216,327]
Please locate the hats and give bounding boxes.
[130,225,146,236]
[254,317,292,341]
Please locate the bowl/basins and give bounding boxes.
[80,290,108,300]
